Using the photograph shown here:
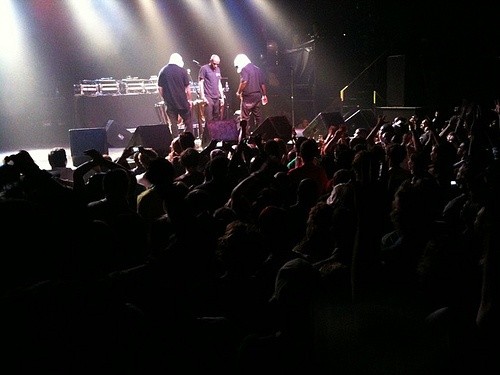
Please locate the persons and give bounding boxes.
[233,54,269,137]
[198,54,224,122]
[0,100,500,375]
[158,53,193,135]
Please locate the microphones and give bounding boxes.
[192,59,200,65]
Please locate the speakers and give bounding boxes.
[345,107,379,132]
[125,125,173,152]
[304,111,346,142]
[68,127,110,164]
[247,114,294,144]
[201,121,238,150]
